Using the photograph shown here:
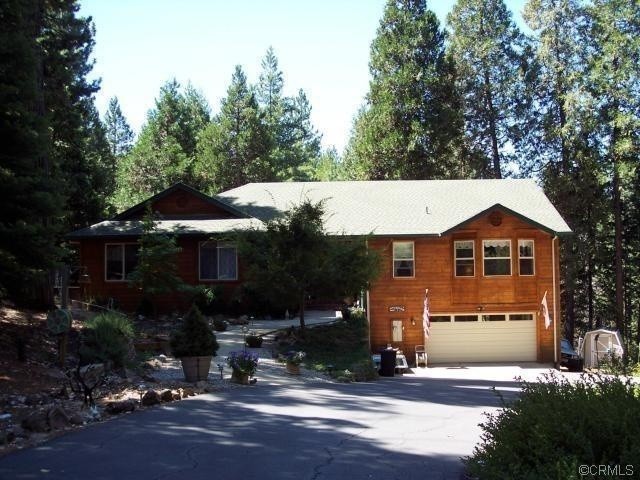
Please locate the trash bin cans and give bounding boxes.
[379,350,397,376]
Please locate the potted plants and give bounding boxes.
[213,320,229,331]
[169,305,219,382]
[245,334,263,348]
[280,295,297,319]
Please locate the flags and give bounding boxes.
[422,294,431,339]
[542,295,551,330]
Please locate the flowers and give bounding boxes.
[287,351,306,363]
[225,350,259,378]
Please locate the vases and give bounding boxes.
[287,363,300,372]
[231,370,250,385]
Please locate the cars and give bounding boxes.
[560,339,579,368]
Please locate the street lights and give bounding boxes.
[57,264,91,367]
